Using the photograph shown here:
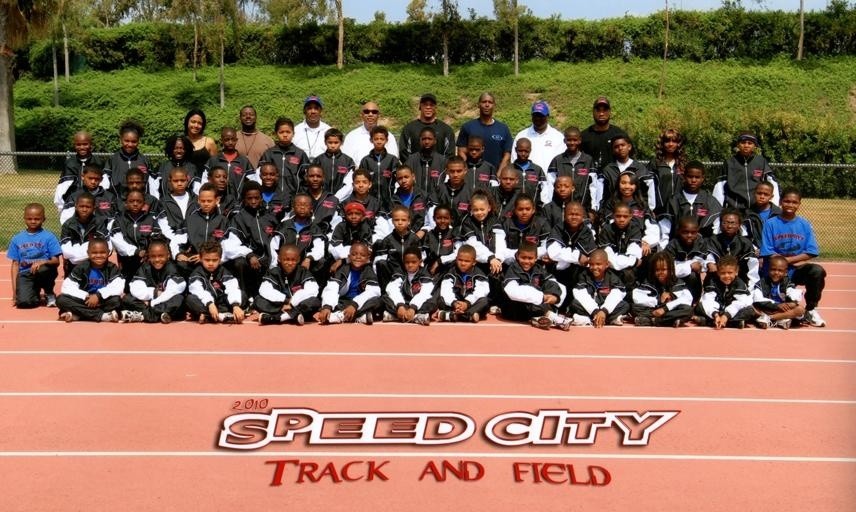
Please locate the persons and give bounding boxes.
[54,122,807,331]
[341,101,400,167]
[291,95,333,159]
[455,91,513,178]
[6,203,63,308]
[510,100,568,178]
[578,97,636,174]
[760,186,827,327]
[184,109,218,177]
[399,94,455,162]
[235,106,276,168]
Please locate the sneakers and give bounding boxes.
[609,309,827,330]
[160,312,173,323]
[46,294,58,307]
[60,311,81,321]
[258,308,480,324]
[199,314,213,323]
[488,305,502,315]
[100,308,144,323]
[530,312,593,329]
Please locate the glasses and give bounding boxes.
[363,109,380,114]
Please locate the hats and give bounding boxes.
[531,99,551,116]
[594,98,612,109]
[420,93,439,106]
[304,95,323,110]
